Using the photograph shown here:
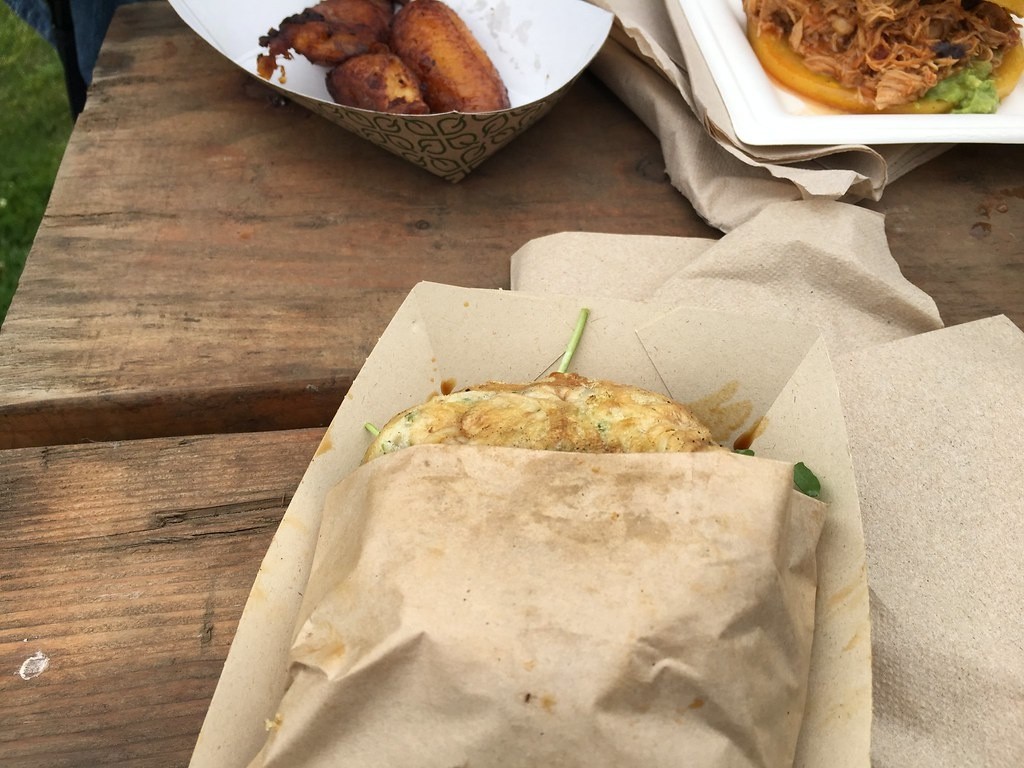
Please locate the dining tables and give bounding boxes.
[0,0,1024,767]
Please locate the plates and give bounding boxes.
[678,0,1024,147]
